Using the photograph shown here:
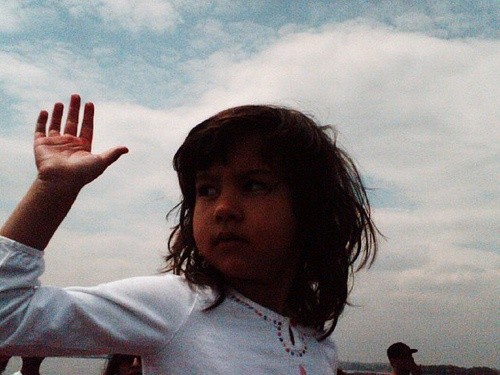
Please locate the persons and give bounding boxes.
[0,349,46,375]
[386,342,425,375]
[0,92,390,375]
[101,354,142,375]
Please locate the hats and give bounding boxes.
[386,342,418,358]
